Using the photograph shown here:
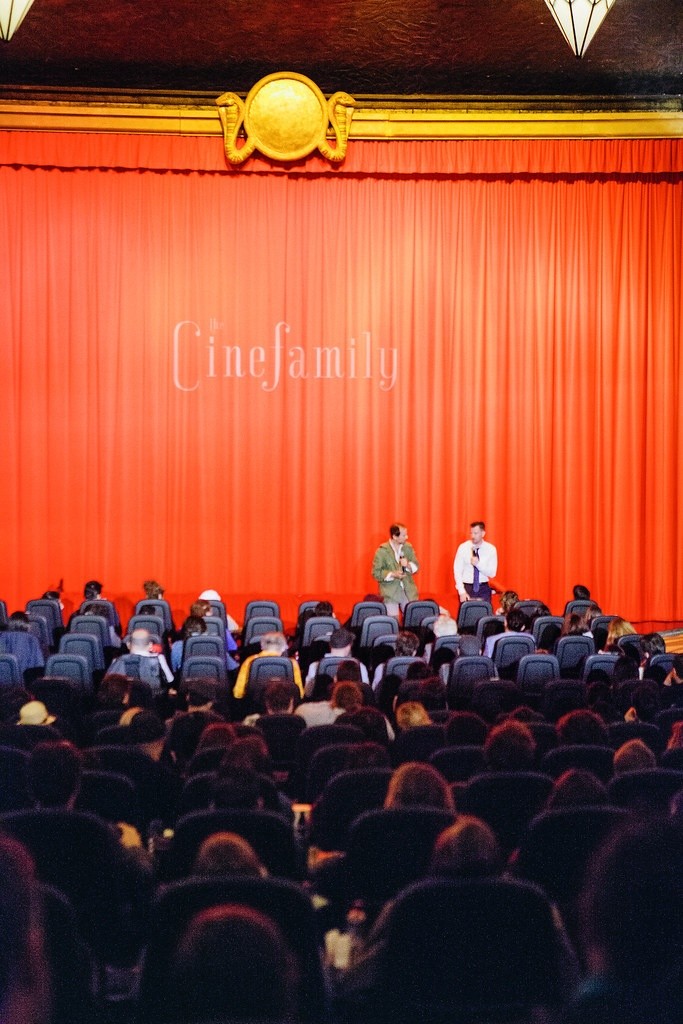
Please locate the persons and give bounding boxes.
[453,521,498,624]
[1,580,683,1024]
[372,523,421,623]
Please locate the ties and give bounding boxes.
[473,549,479,593]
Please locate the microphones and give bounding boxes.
[400,552,406,572]
[473,544,476,557]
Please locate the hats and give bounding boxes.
[17,701,55,725]
[199,590,220,601]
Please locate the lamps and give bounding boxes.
[544,0,617,60]
[0,0,35,42]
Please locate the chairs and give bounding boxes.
[1,598,683,1024]
